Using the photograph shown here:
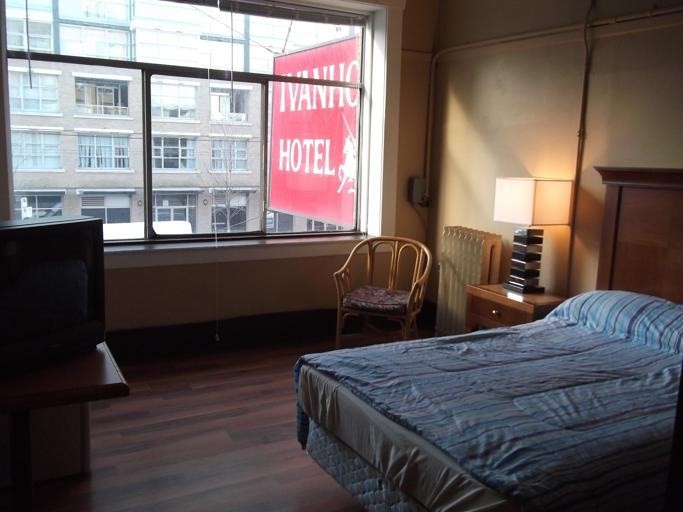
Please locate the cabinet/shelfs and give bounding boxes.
[0,331,133,491]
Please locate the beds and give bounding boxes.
[291,161,682,512]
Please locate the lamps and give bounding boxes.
[491,171,574,296]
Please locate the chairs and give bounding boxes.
[330,232,431,346]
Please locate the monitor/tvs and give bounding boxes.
[0,213,108,372]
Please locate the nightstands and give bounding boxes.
[461,281,564,331]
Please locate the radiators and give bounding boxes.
[430,222,501,338]
